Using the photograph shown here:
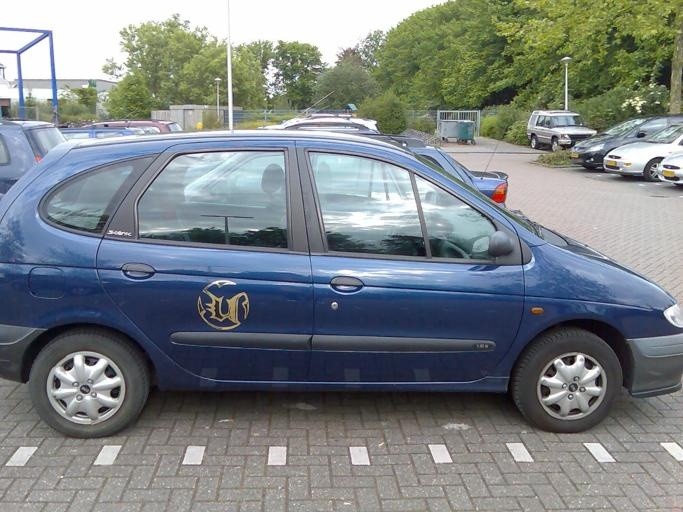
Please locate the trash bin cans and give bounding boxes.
[457,120,476,145]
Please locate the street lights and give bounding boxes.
[559,55,572,112]
[214,77,221,115]
